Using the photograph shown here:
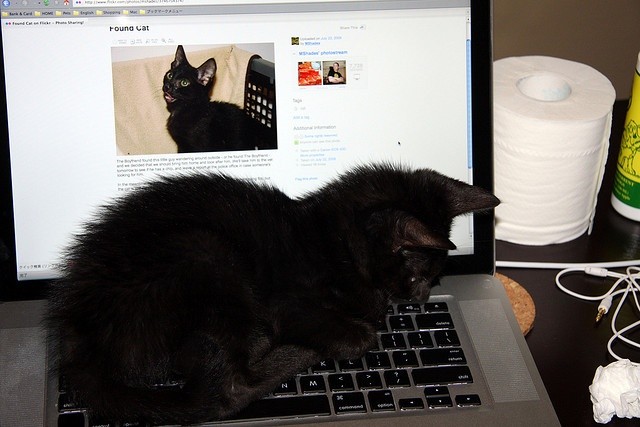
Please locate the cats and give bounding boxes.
[162,44,277,153]
[41,160,502,427]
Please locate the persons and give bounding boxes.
[327,62,345,84]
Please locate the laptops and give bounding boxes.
[1,1,562,425]
